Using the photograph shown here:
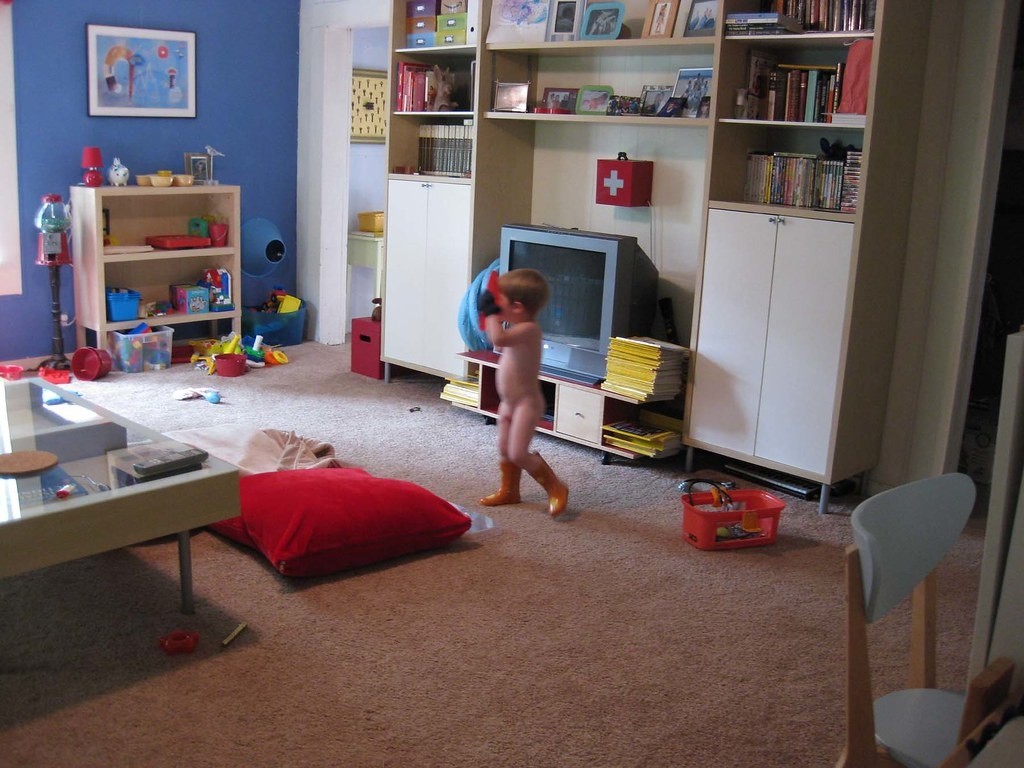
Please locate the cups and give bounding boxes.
[156,170,173,177]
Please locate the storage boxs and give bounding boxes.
[244,299,308,346]
[678,485,778,549]
[594,152,655,210]
[350,316,388,379]
[406,33,436,48]
[441,1,468,15]
[357,211,383,237]
[104,285,141,321]
[407,1,437,18]
[107,326,175,372]
[167,283,210,314]
[436,31,466,46]
[437,15,467,31]
[405,16,436,33]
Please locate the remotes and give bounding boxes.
[132,449,208,474]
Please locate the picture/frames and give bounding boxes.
[540,86,580,114]
[640,85,673,115]
[641,0,682,38]
[84,21,198,120]
[680,0,719,37]
[351,68,388,144]
[656,97,687,117]
[184,153,211,185]
[575,84,615,116]
[584,2,626,40]
[672,67,712,118]
[492,80,530,112]
[696,96,710,118]
[545,0,587,40]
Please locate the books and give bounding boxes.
[742,150,863,213]
[600,336,691,402]
[724,0,878,35]
[600,420,683,459]
[418,123,473,178]
[440,369,479,408]
[746,54,867,127]
[395,60,455,112]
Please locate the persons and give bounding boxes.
[688,6,715,31]
[654,3,667,34]
[610,96,640,115]
[548,92,576,112]
[480,268,570,516]
[584,93,608,111]
[682,72,708,113]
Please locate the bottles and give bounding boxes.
[34,193,72,232]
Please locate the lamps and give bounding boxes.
[79,145,106,187]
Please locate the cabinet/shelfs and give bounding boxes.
[685,0,935,513]
[483,0,723,126]
[68,182,246,370]
[378,0,538,391]
[450,349,684,469]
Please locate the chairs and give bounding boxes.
[841,471,1024,768]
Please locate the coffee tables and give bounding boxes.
[0,375,246,615]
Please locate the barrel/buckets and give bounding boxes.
[71,347,112,382]
[209,224,228,247]
[214,354,246,376]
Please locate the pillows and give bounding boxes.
[205,514,258,553]
[239,467,473,581]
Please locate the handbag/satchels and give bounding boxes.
[837,38,872,115]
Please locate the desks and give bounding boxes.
[348,231,381,316]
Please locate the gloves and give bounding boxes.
[479,289,500,317]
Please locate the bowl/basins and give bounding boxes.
[174,175,194,186]
[136,174,151,187]
[149,176,173,187]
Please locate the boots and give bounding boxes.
[528,452,568,515]
[480,461,521,505]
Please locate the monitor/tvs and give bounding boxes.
[493,225,659,385]
